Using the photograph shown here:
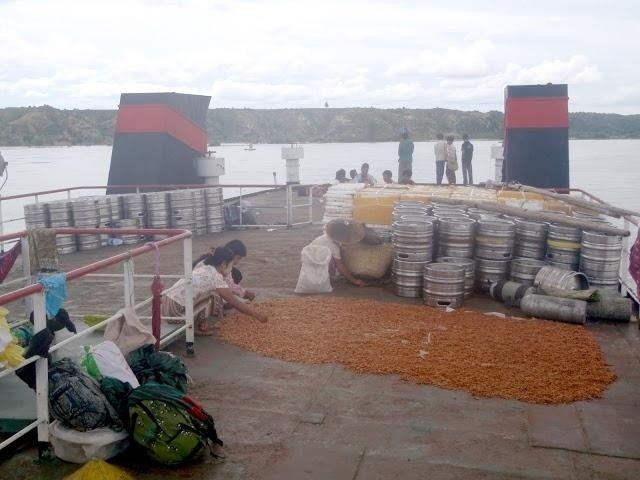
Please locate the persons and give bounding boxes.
[397,133,414,174]
[461,133,473,185]
[433,134,446,185]
[336,169,348,183]
[358,163,377,186]
[447,136,458,184]
[308,218,365,289]
[162,248,269,337]
[193,239,257,311]
[382,169,396,184]
[350,169,357,179]
[397,170,416,185]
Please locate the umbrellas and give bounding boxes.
[144,241,164,351]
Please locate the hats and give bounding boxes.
[326,218,366,244]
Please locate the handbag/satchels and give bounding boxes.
[48,344,208,467]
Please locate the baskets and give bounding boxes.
[341,244,392,280]
